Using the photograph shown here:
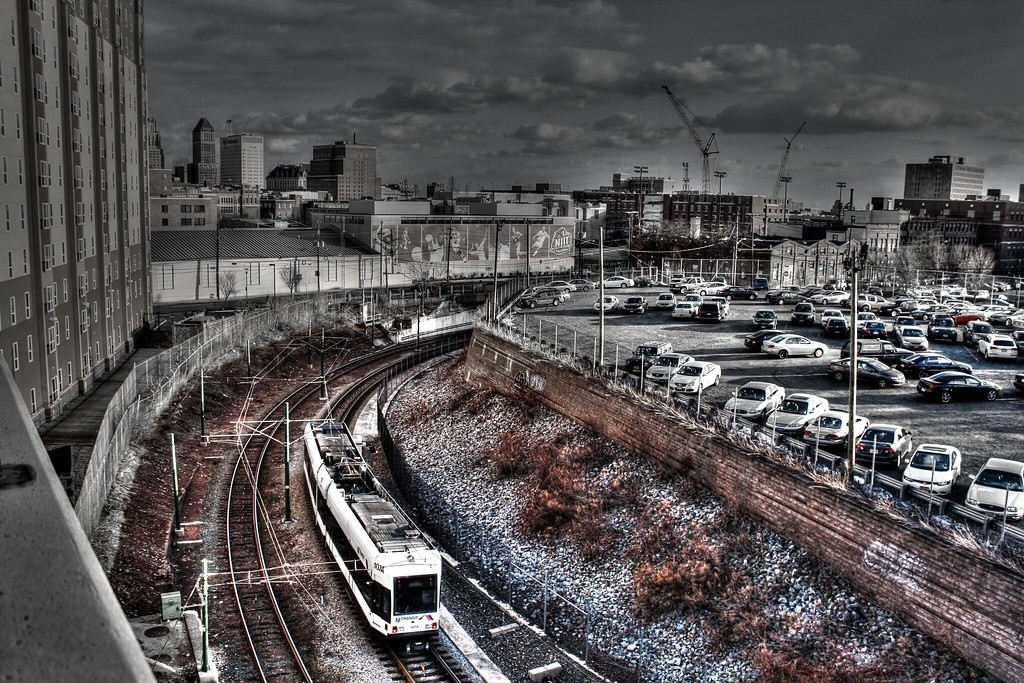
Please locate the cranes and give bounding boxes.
[773,121,806,199]
[661,83,720,195]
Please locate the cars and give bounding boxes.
[723,381,786,423]
[751,309,777,330]
[896,325,929,351]
[744,329,787,351]
[902,444,962,496]
[645,353,695,383]
[855,424,913,467]
[977,334,1018,361]
[669,361,722,395]
[1010,331,1024,352]
[827,356,906,389]
[916,371,1004,404]
[761,334,829,360]
[766,392,830,435]
[520,274,1024,340]
[962,320,999,347]
[901,352,973,379]
[803,411,870,451]
[966,457,1024,521]
[860,321,888,340]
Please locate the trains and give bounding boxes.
[303,422,441,655]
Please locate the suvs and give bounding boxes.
[927,314,957,343]
[841,339,916,365]
[625,340,673,374]
